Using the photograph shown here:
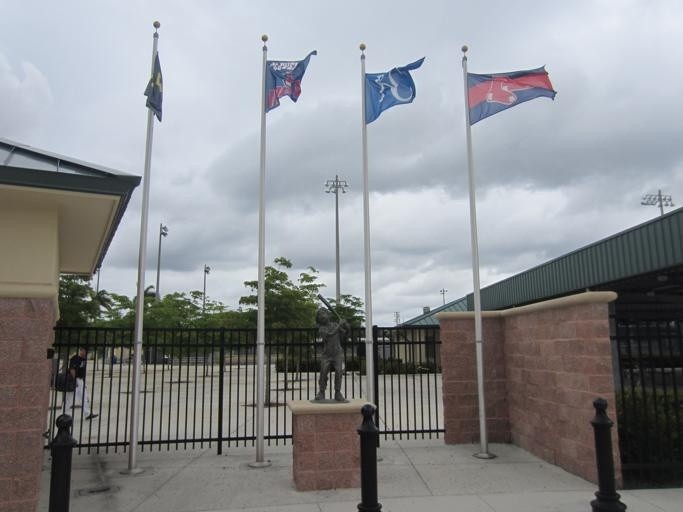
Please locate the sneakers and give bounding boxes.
[86,413,100,419]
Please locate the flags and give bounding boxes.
[366,55,427,126]
[265,50,317,112]
[467,66,557,127]
[144,51,164,123]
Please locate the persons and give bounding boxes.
[60,345,101,421]
[314,306,353,403]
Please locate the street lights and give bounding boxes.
[639,190,674,218]
[155,222,169,304]
[202,263,210,314]
[323,174,349,307]
